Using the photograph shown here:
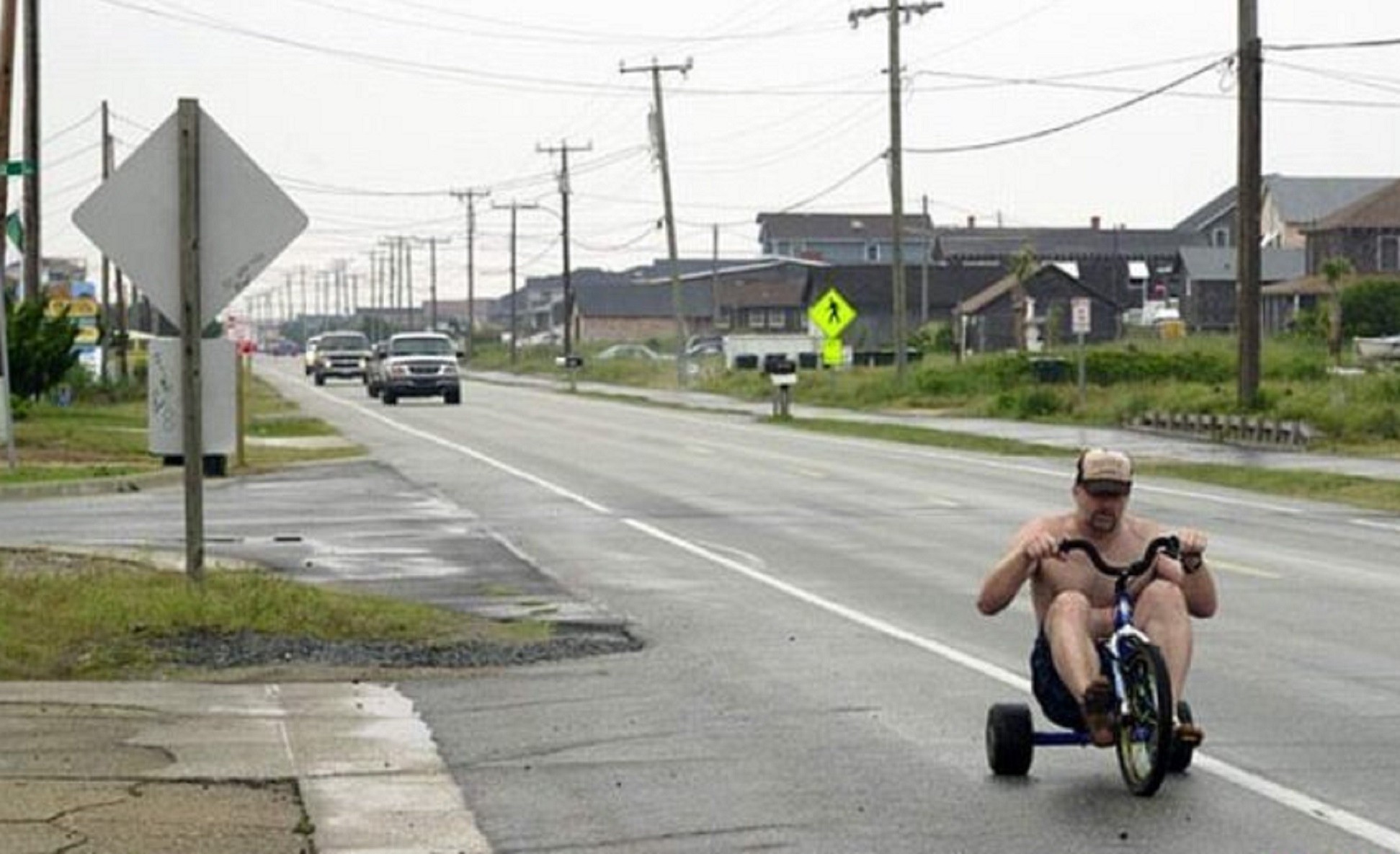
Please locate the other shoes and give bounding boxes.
[1083,680,1118,748]
[1171,722,1205,749]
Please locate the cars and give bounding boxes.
[362,341,391,397]
[594,342,678,361]
[269,339,300,358]
[303,336,319,375]
[683,335,726,358]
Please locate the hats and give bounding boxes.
[1076,448,1132,492]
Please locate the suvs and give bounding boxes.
[378,331,466,406]
[311,329,376,386]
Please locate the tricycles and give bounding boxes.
[984,532,1206,800]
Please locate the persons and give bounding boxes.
[978,447,1219,747]
